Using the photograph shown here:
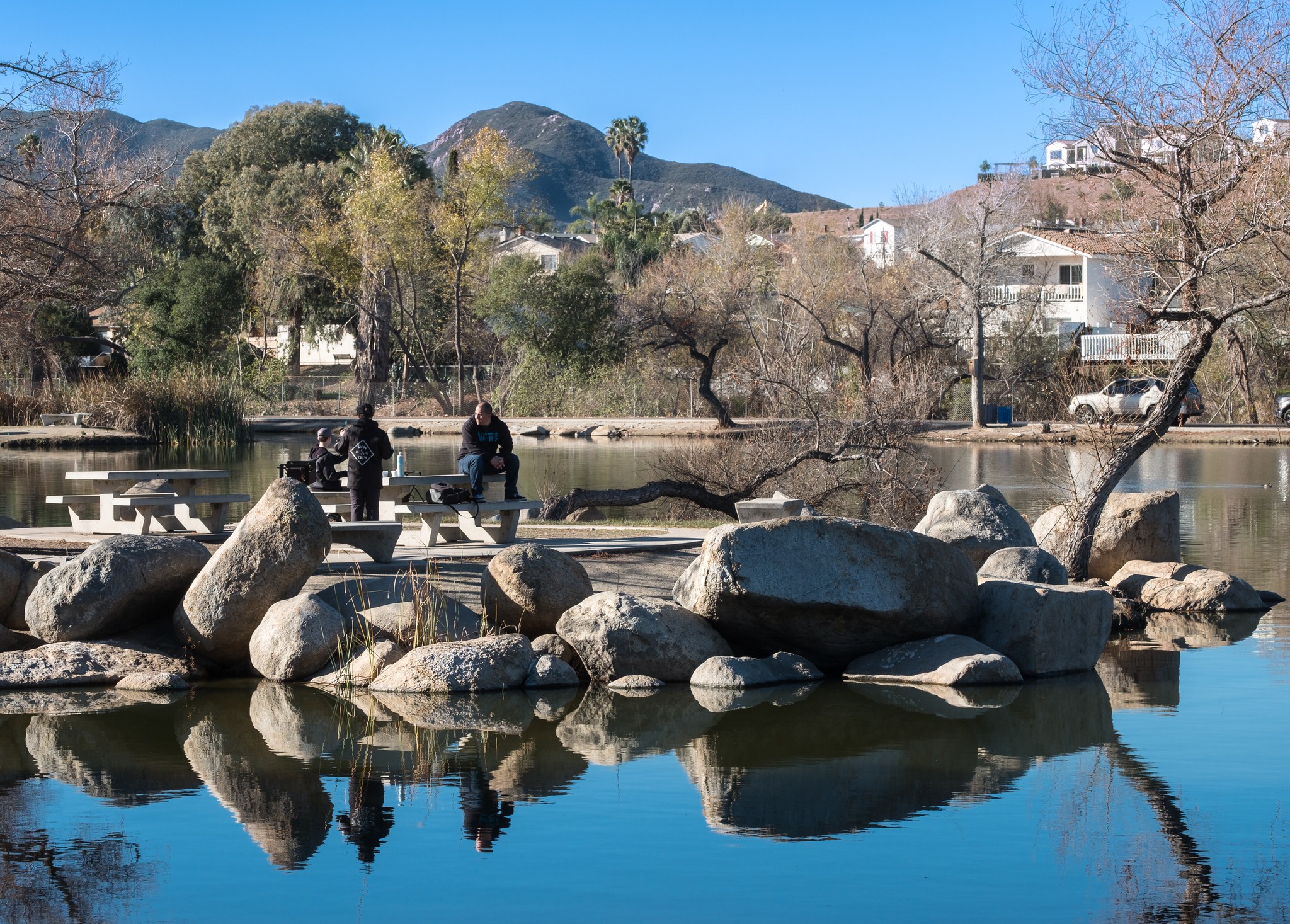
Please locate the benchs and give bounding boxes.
[380,473,543,547]
[39,414,83,425]
[333,354,355,364]
[734,498,805,526]
[308,491,352,522]
[46,468,250,535]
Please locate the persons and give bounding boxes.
[334,403,394,522]
[306,427,351,492]
[456,402,528,504]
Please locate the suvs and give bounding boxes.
[1068,374,1204,426]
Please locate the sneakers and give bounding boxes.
[326,512,341,520]
[504,493,527,501]
[474,494,487,502]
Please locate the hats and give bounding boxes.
[355,403,374,415]
[318,427,334,438]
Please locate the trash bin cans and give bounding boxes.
[998,406,1012,424]
[983,404,998,424]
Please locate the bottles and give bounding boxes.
[397,452,405,476]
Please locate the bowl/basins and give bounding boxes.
[391,470,398,477]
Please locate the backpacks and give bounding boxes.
[426,482,479,518]
